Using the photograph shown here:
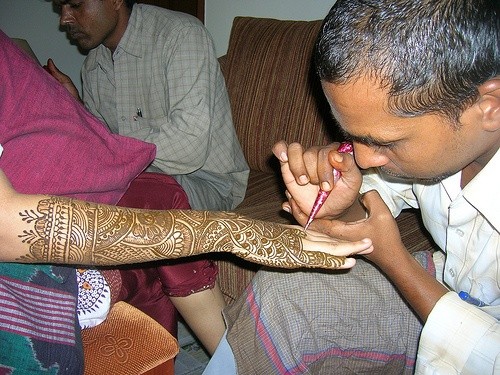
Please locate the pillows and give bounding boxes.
[0,28,158,208]
[225,17,341,172]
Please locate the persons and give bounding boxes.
[0,29,373,375]
[201,0,500,375]
[43,0,250,214]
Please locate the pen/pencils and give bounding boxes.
[458,291,490,307]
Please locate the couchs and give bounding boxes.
[217,16,439,305]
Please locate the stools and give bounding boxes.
[81,300,180,375]
